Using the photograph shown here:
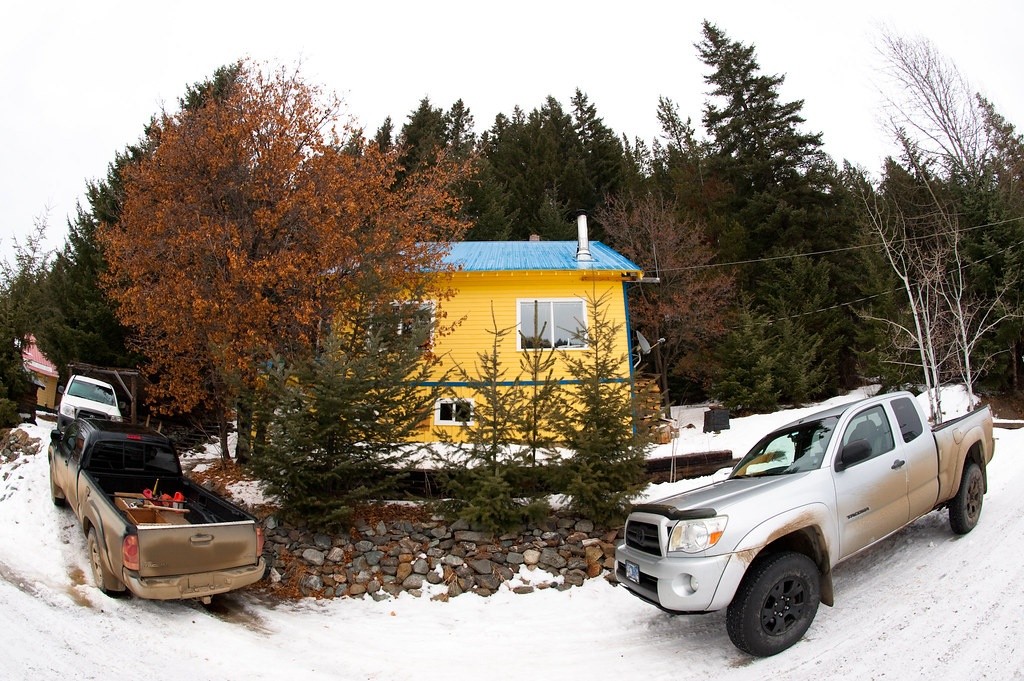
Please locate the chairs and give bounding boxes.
[819,431,844,461]
[856,421,886,451]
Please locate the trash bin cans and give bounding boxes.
[703,408,730,433]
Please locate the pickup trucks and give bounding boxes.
[611,388,996,656]
[47,417,267,604]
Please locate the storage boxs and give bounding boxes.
[154,510,192,524]
[125,508,172,526]
[114,492,154,510]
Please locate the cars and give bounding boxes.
[56,374,122,432]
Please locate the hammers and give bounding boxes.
[137,503,190,513]
